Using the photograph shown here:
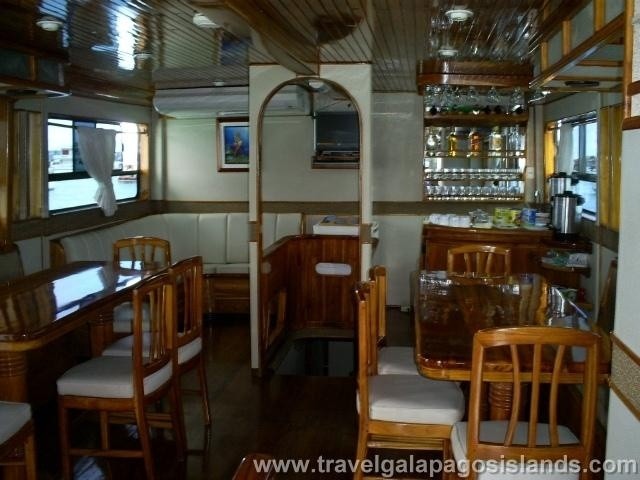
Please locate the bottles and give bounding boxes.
[425,123,523,155]
[431,104,523,117]
[424,167,523,199]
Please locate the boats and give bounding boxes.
[47,146,123,177]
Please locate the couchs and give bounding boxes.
[51,213,304,313]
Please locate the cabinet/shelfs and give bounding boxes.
[416,72,531,203]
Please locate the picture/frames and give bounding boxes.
[212,28,250,65]
[215,117,250,173]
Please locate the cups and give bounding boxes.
[429,213,471,229]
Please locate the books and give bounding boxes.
[312,216,379,238]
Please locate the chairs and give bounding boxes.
[0,236,600,480]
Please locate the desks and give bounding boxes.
[419,221,592,304]
